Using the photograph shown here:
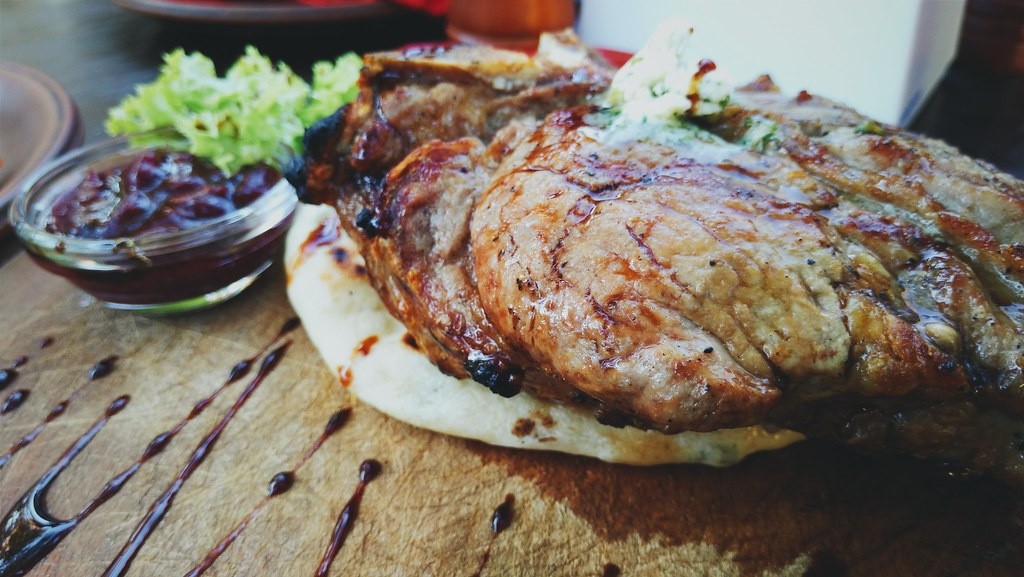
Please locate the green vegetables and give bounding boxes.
[100,42,367,181]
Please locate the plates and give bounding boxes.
[0,59,76,233]
[108,0,399,29]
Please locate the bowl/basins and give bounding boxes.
[8,132,300,318]
